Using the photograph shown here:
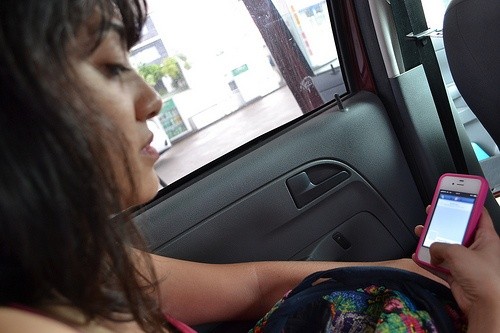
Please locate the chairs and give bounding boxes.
[442,0,499,151]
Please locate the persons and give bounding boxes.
[0,0,500,333]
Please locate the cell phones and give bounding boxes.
[415,173,488,272]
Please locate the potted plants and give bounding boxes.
[137,63,169,96]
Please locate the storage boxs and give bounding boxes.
[188,103,226,130]
